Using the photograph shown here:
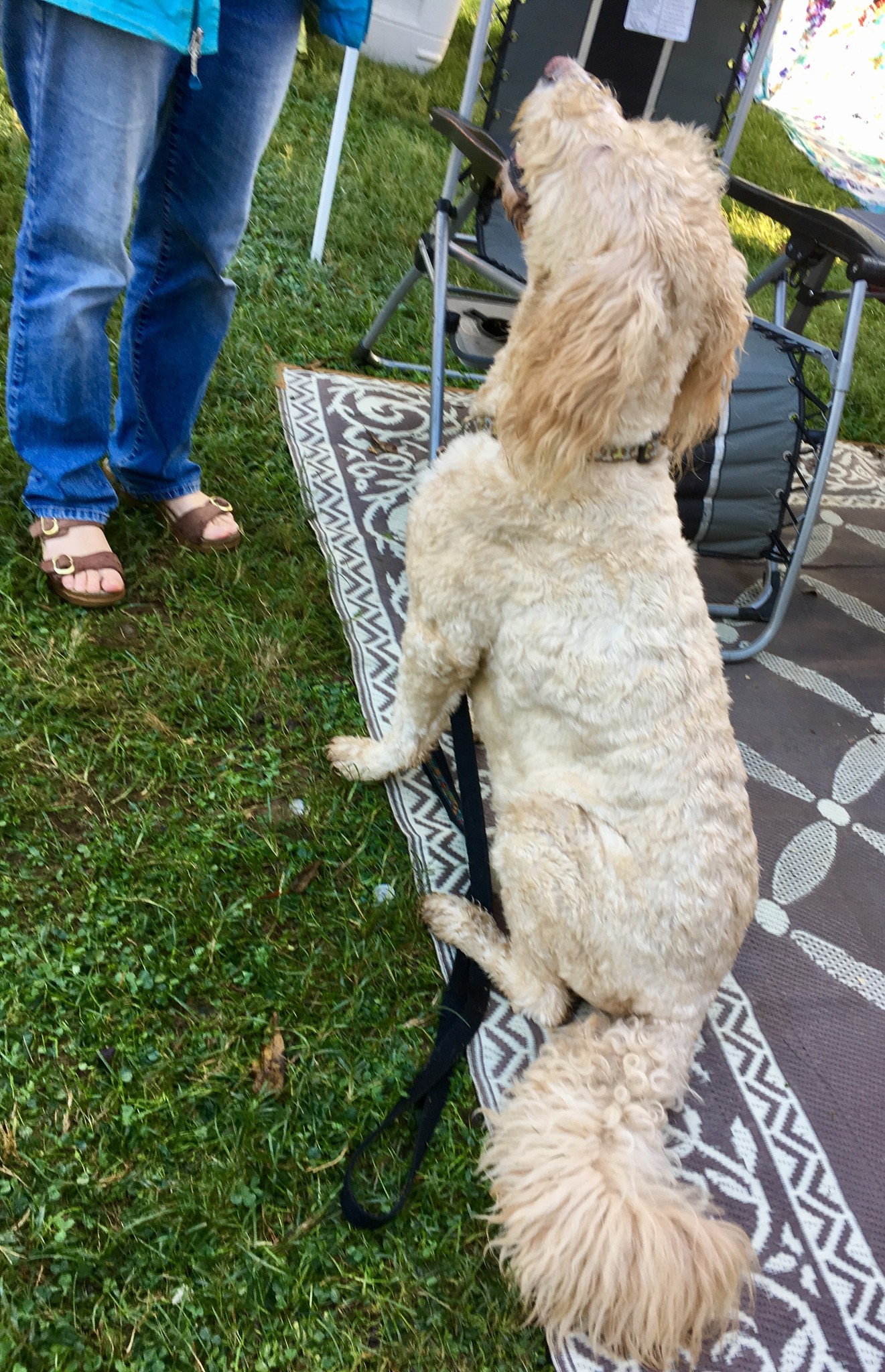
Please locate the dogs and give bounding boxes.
[324,51,762,1369]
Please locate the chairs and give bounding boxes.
[354,0,885,663]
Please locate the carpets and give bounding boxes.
[277,361,884,1371]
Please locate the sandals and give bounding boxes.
[28,516,126,607]
[126,489,242,554]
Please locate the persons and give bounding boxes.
[0,1,374,609]
